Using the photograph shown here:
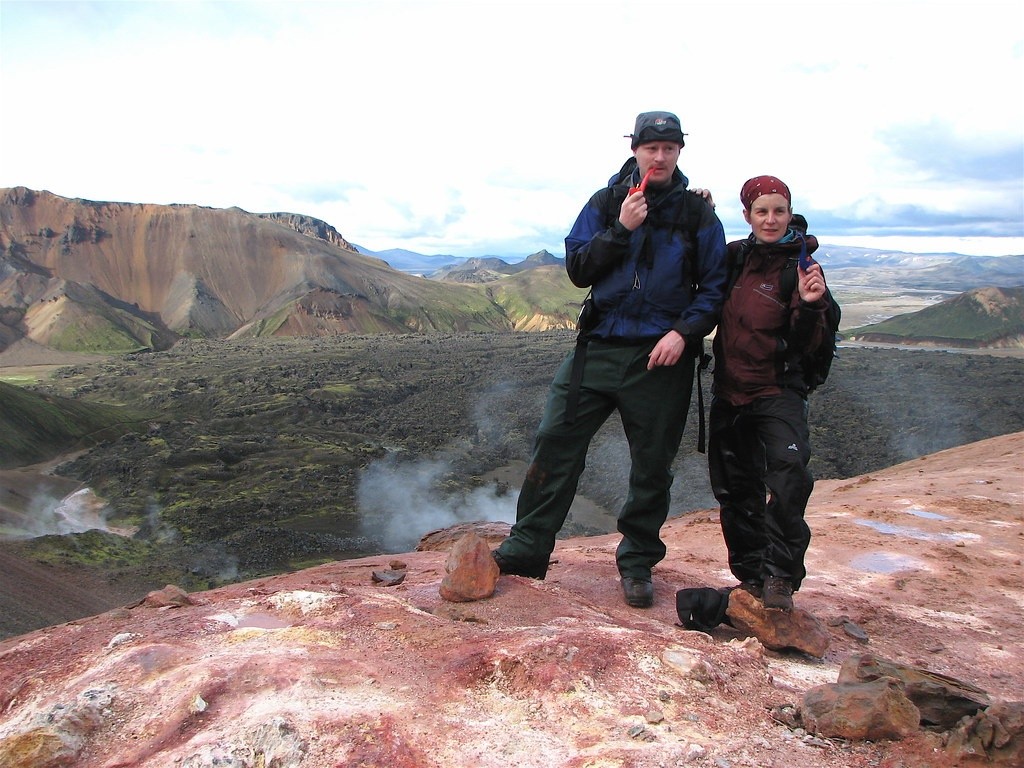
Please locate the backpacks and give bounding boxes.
[724,214,841,387]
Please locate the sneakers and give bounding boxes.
[761,580,794,612]
[621,576,653,607]
[491,550,525,576]
[718,582,761,598]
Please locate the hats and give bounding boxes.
[631,112,684,150]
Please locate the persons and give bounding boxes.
[688,175,830,606]
[490,111,726,606]
